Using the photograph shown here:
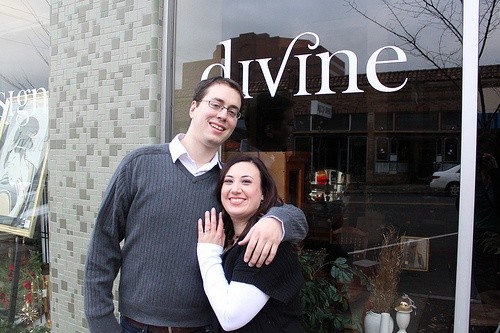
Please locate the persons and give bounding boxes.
[240,91,296,154]
[197,154,305,333]
[84,76,310,333]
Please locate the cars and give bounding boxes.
[430,164,460,197]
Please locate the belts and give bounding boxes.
[123,315,204,333]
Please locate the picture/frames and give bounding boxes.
[400,236,430,272]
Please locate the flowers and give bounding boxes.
[0,251,39,311]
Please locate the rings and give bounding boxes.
[205,228,212,233]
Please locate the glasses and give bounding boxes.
[197,100,241,119]
